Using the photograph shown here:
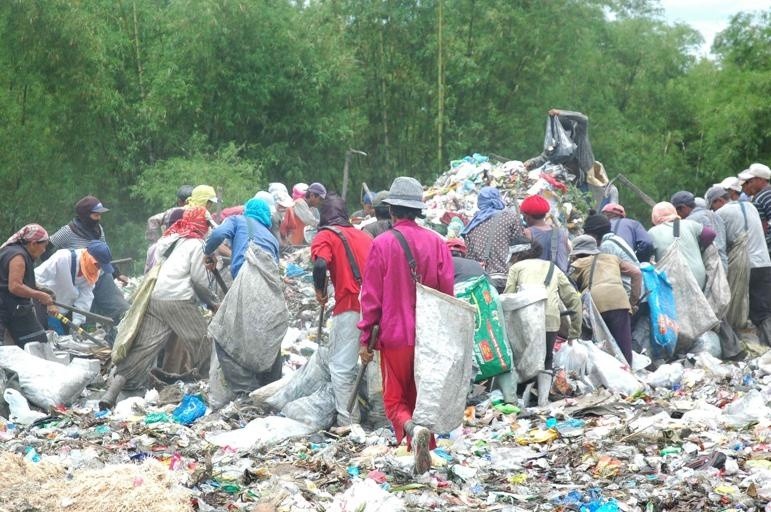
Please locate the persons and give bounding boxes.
[524,108,596,195]
[1,223,58,349]
[101,162,771,437]
[34,240,114,335]
[359,176,455,472]
[42,196,130,323]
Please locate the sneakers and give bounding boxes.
[328,422,366,448]
[410,425,432,474]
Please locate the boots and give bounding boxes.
[537,370,554,407]
[99,373,127,410]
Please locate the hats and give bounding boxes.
[520,194,551,217]
[86,240,116,274]
[76,196,111,214]
[305,183,329,200]
[672,162,771,209]
[569,201,627,256]
[364,177,428,209]
